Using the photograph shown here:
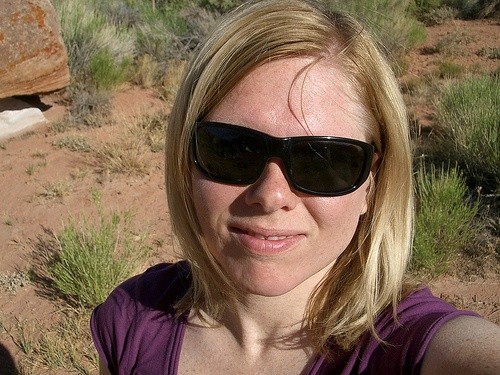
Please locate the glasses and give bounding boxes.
[190,121,375,197]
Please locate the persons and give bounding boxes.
[87,1,500,375]
